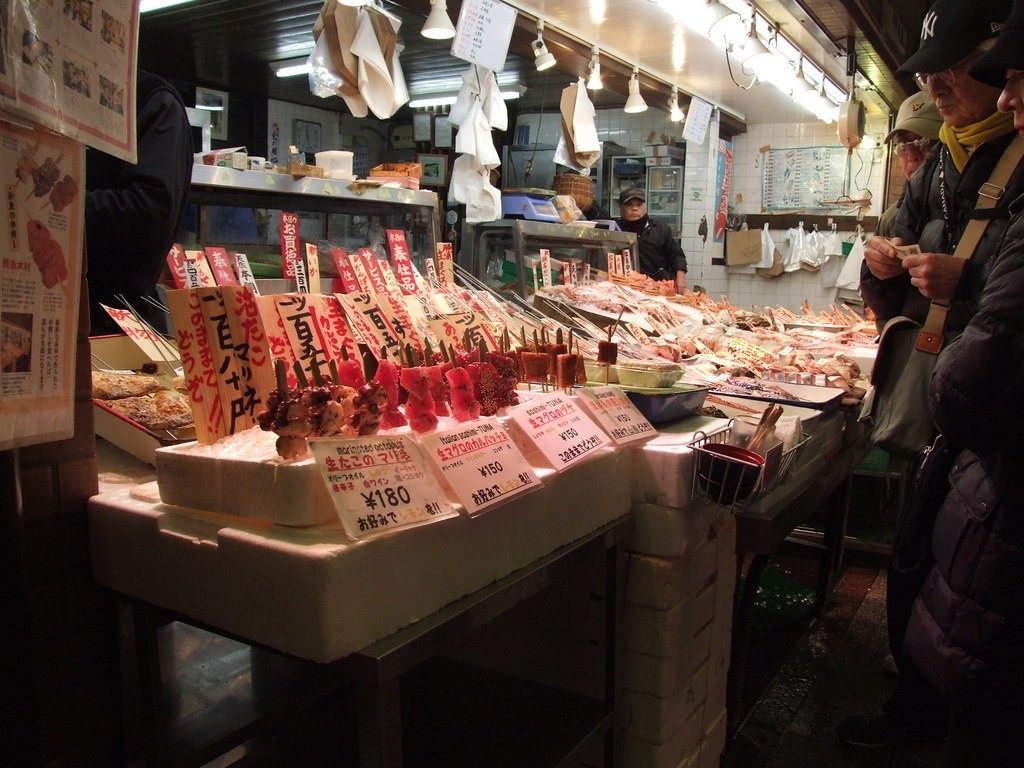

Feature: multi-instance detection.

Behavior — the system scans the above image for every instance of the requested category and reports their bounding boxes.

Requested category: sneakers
[836,706,951,748]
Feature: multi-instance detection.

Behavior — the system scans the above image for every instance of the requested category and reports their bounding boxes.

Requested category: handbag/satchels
[857,316,960,460]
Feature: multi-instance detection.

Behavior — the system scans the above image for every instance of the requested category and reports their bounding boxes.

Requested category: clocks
[292,118,322,154]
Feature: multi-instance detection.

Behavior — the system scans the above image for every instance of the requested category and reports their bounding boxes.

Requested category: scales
[820,93,871,206]
[501,187,561,222]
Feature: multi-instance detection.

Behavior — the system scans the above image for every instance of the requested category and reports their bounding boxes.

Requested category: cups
[729,415,776,455]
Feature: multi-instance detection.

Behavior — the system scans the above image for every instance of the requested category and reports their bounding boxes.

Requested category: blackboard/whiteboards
[450,0,518,73]
[682,96,713,146]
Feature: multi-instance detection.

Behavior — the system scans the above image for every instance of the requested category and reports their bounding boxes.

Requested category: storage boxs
[614,164,645,176]
[87,390,847,768]
[646,144,685,167]
[315,150,354,181]
[194,146,248,170]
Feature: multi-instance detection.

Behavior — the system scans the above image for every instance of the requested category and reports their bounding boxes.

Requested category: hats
[620,188,645,203]
[969,0,1024,89]
[883,90,945,144]
[894,0,1013,81]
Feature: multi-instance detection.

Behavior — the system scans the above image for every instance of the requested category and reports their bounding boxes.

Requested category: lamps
[421,1,457,40]
[586,45,603,90]
[667,85,684,122]
[530,19,557,71]
[705,0,840,123]
[624,66,648,114]
[407,85,528,107]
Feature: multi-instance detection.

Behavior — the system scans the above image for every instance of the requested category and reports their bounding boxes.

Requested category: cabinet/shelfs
[648,166,684,232]
[611,156,648,217]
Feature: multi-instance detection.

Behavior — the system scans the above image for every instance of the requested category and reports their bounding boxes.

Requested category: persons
[599,186,688,295]
[860,3,1013,747]
[899,1,1024,767]
[874,91,946,339]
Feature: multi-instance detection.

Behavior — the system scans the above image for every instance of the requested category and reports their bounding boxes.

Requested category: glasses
[913,50,984,94]
[893,140,936,156]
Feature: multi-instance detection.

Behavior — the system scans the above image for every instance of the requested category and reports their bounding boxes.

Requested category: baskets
[551,174,593,212]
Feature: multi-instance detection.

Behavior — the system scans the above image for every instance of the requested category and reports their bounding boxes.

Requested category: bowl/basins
[315,150,354,179]
[699,443,765,506]
[583,361,618,385]
[610,364,686,388]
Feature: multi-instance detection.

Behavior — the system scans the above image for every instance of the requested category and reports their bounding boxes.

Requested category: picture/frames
[417,154,448,186]
[195,86,229,142]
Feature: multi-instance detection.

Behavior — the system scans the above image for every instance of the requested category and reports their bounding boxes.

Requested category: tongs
[747,402,784,453]
